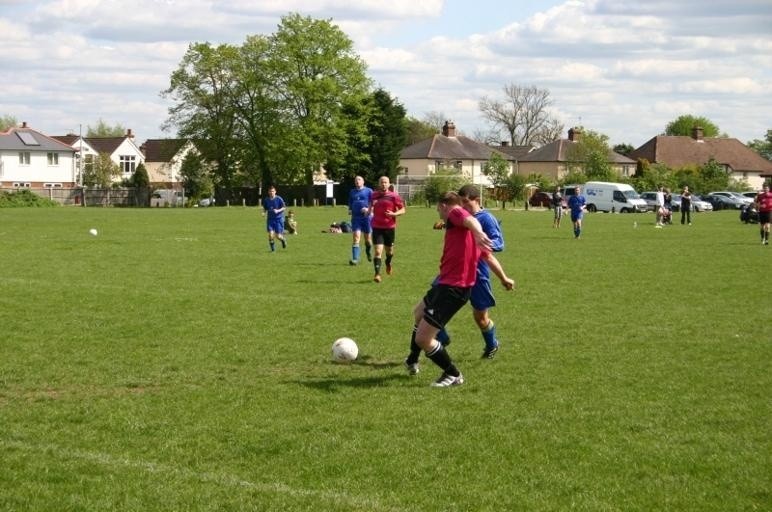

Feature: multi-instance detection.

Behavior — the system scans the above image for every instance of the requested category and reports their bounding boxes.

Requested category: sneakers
[385,260,392,274]
[482,341,498,358]
[403,356,418,375]
[374,275,382,282]
[431,371,464,387]
[283,238,287,248]
[368,253,373,261]
[349,260,358,265]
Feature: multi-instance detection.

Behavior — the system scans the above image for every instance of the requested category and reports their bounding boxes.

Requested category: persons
[367,176,406,283]
[348,175,374,265]
[553,185,565,228]
[757,182,772,245]
[654,184,692,228]
[262,186,287,251]
[284,209,297,235]
[402,191,515,389]
[431,185,502,359]
[564,186,587,240]
[744,196,760,224]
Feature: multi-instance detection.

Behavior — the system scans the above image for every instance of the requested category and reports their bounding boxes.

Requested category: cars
[151,190,215,208]
[530,192,554,207]
[640,191,762,212]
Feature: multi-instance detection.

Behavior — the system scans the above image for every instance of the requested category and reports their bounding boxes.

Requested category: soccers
[89,229,98,237]
[332,338,358,363]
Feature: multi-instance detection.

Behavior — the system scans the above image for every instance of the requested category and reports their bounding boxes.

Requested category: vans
[559,186,575,209]
[583,181,648,214]
